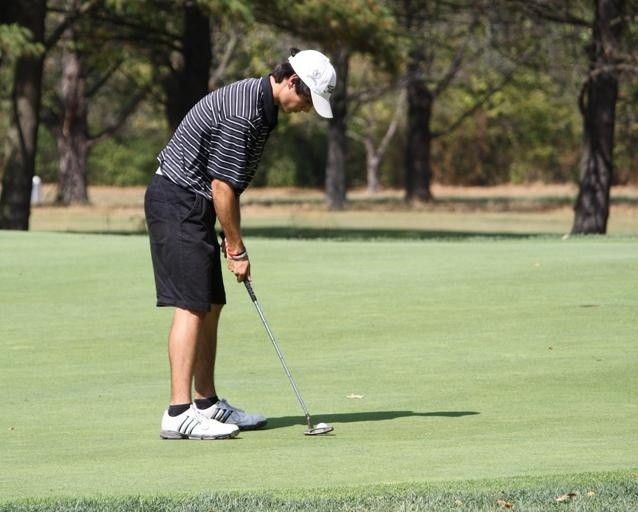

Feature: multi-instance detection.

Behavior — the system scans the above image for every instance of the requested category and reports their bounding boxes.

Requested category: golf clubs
[220,231,334,435]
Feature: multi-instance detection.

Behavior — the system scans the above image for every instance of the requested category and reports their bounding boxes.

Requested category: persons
[144,48,337,442]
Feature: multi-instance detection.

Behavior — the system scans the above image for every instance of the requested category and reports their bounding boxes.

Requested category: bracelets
[229,252,247,259]
[227,248,244,257]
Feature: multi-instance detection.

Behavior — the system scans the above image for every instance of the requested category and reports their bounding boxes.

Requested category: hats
[287,49,336,121]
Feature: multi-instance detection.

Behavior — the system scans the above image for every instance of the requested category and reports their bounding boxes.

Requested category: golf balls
[317,423,327,428]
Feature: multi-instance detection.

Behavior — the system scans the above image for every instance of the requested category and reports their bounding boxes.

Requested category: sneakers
[158,402,242,441]
[192,398,267,431]
